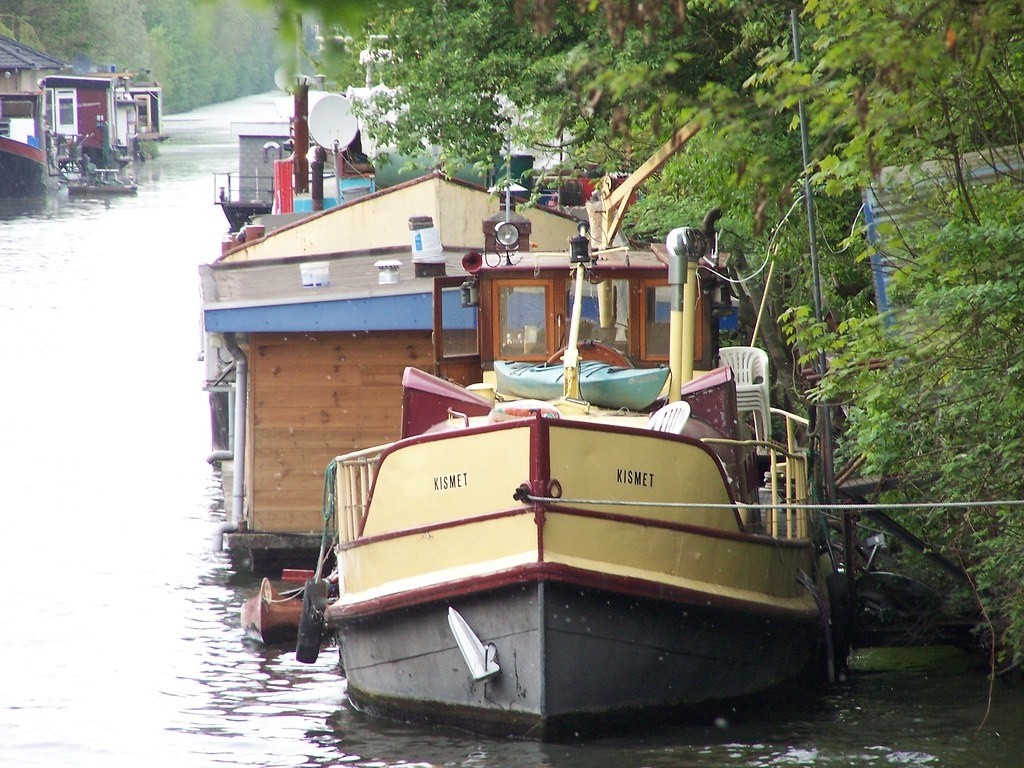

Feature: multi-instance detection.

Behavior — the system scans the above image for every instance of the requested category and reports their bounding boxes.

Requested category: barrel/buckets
[412,228,442,259]
[299,261,330,289]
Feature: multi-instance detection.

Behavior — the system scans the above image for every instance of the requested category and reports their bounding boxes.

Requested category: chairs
[718,346,771,448]
[643,401,690,434]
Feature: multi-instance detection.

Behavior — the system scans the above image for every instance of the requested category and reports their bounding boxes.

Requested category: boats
[494,355,673,415]
[240,579,306,641]
[65,168,138,194]
[287,103,852,729]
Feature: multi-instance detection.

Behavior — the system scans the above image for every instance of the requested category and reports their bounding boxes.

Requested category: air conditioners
[205,331,236,383]
